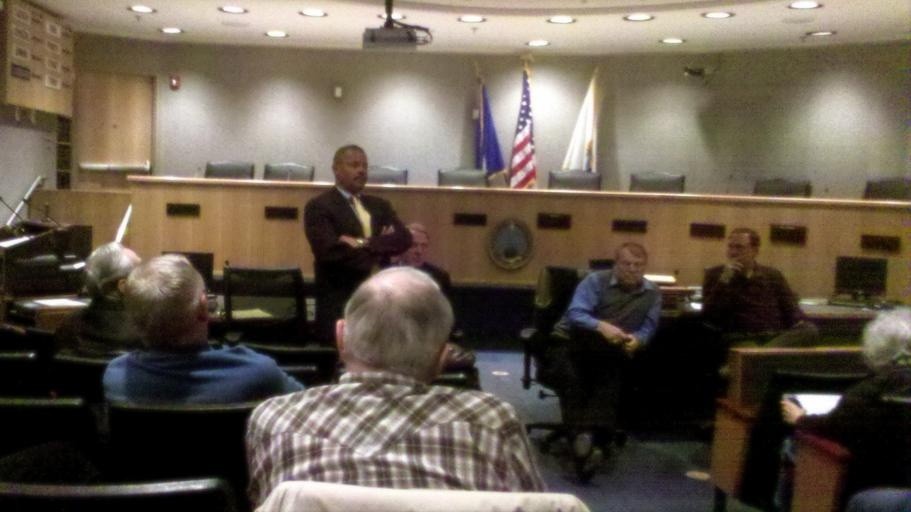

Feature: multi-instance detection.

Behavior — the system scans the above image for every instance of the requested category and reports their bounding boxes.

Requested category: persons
[779,304,911,511]
[103,251,308,404]
[244,265,571,512]
[54,241,142,359]
[546,242,662,485]
[304,145,411,375]
[698,227,818,384]
[394,222,456,322]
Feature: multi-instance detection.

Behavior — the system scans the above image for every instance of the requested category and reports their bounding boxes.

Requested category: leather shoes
[572,431,592,459]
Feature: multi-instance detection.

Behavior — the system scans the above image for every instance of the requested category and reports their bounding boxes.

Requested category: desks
[787,426,858,511]
[797,305,878,343]
[711,398,762,512]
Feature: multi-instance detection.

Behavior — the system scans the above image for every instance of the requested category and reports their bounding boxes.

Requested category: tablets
[778,390,843,424]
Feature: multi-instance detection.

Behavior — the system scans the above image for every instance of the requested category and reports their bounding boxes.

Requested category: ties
[575,448,603,486]
[351,196,372,239]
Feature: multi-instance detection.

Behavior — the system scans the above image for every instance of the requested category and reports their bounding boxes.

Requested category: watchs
[356,238,365,249]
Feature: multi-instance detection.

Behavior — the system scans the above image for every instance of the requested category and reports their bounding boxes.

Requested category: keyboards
[827,297,882,311]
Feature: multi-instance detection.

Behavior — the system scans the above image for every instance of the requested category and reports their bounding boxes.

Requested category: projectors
[360,26,418,47]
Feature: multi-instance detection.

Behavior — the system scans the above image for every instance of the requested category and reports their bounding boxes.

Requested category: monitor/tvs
[834,256,889,298]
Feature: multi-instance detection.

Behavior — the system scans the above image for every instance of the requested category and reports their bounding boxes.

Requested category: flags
[509,62,537,189]
[560,65,601,174]
[475,76,505,175]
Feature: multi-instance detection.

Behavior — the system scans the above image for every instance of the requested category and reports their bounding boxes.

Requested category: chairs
[223,267,308,343]
[0,395,106,483]
[366,166,410,185]
[733,369,873,510]
[264,162,315,182]
[548,171,603,189]
[753,180,812,197]
[0,476,235,512]
[521,264,637,459]
[204,162,254,177]
[53,352,108,397]
[1,353,42,393]
[863,177,910,199]
[161,250,214,289]
[256,480,589,512]
[106,399,255,506]
[630,172,687,191]
[439,169,488,187]
[2,323,64,362]
